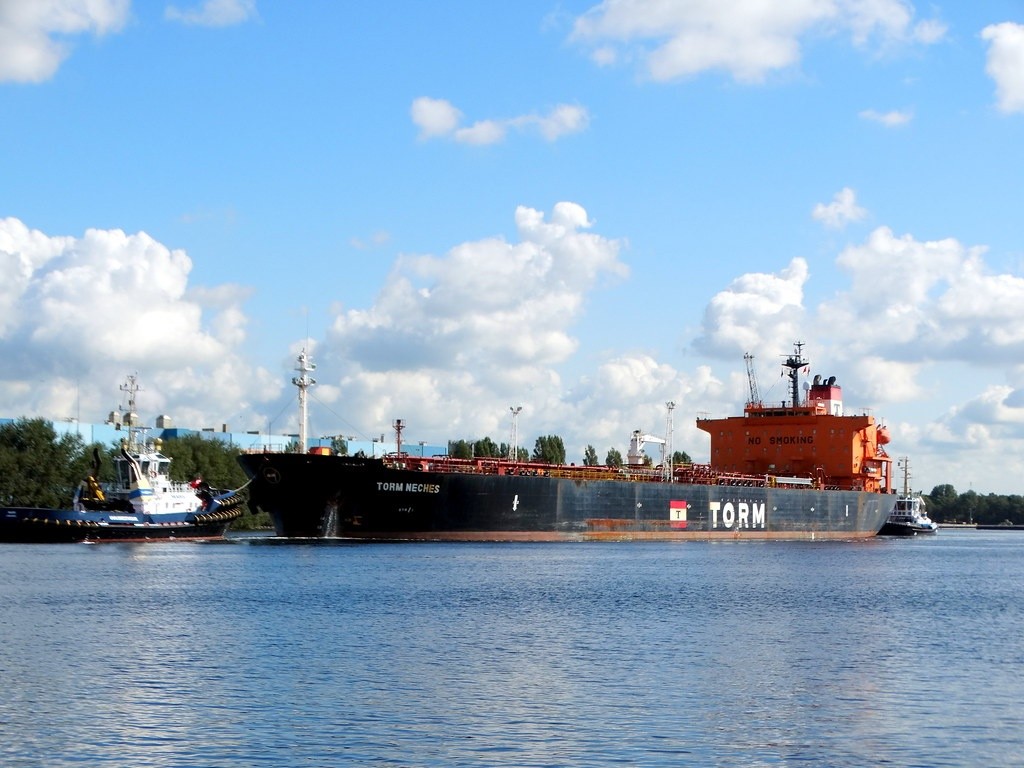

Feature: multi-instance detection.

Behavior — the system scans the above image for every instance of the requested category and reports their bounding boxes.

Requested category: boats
[882,487,939,534]
[0,375,243,540]
[232,337,901,544]
